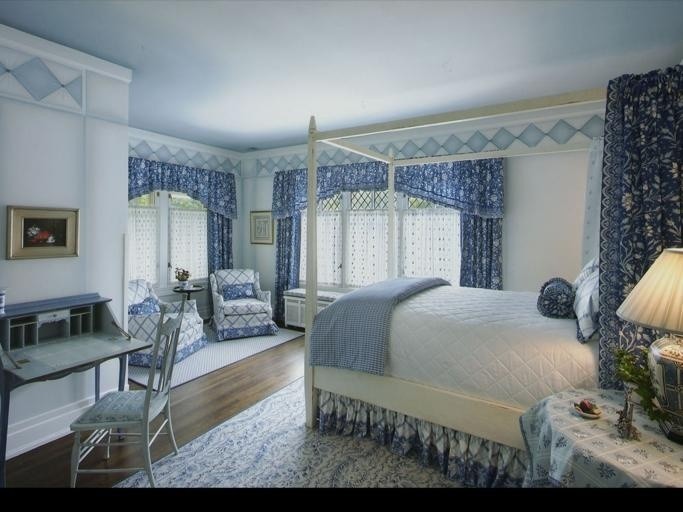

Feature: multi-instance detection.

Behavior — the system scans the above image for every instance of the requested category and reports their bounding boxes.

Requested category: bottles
[0,289,6,316]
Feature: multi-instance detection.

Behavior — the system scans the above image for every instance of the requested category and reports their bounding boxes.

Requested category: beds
[306,82,606,489]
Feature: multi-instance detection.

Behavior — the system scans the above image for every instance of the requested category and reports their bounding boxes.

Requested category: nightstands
[519,387,683,488]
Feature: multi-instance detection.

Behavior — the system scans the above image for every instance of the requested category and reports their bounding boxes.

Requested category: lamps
[617,247,683,444]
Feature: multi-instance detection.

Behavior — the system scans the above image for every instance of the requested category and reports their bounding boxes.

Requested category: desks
[0,292,153,488]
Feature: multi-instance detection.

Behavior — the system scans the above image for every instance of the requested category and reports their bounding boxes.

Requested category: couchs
[128,278,208,369]
[209,269,279,342]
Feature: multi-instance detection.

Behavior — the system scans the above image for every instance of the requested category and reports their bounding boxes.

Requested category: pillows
[128,297,161,315]
[537,255,601,343]
[222,283,257,300]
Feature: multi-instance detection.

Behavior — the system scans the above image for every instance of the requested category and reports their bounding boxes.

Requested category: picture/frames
[250,210,274,244]
[6,204,79,260]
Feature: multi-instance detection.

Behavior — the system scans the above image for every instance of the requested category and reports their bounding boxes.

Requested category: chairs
[68,300,183,487]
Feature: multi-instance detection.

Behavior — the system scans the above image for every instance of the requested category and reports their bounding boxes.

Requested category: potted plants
[175,267,191,289]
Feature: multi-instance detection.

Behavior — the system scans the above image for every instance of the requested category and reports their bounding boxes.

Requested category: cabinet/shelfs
[283,295,333,329]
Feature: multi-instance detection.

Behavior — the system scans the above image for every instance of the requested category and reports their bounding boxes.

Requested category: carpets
[114,377,483,488]
[127,322,304,392]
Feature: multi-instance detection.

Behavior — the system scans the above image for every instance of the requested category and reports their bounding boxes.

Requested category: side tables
[173,285,204,300]
